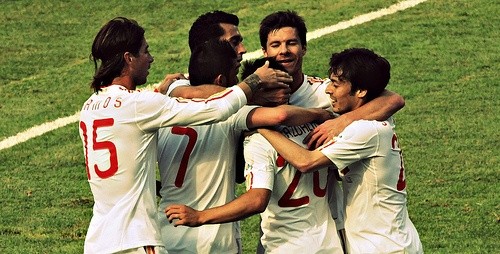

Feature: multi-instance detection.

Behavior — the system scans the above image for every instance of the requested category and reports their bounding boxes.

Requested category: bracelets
[243,74,264,98]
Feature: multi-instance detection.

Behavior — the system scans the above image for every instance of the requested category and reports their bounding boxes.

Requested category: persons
[156,39,336,254]
[165,10,247,184]
[79,16,293,254]
[243,48,424,254]
[164,56,344,254]
[257,9,405,254]
